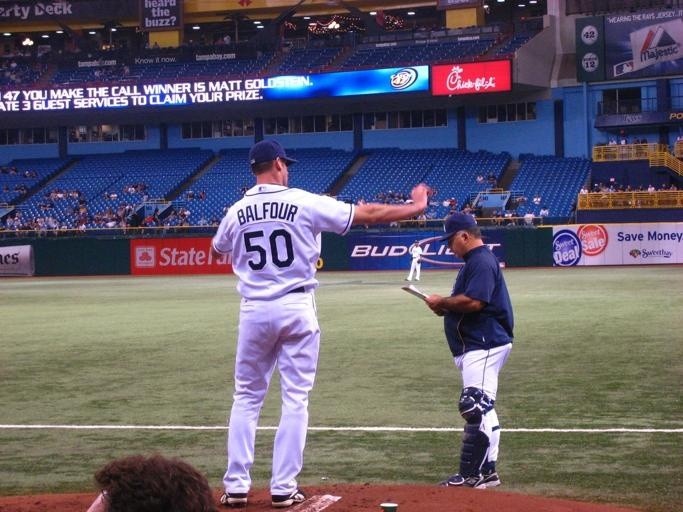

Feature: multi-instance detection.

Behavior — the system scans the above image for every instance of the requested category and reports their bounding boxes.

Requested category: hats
[249,139,300,168]
[439,213,478,242]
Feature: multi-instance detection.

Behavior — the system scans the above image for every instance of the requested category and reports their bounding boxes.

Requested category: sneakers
[271,491,306,507]
[220,492,248,507]
[439,473,502,489]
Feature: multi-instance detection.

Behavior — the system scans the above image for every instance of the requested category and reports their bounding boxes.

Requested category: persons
[424,215,515,489]
[211,139,431,508]
[476,172,496,184]
[356,191,548,228]
[580,181,678,205]
[0,166,249,238]
[405,240,423,280]
[85,455,217,511]
[608,136,648,145]
